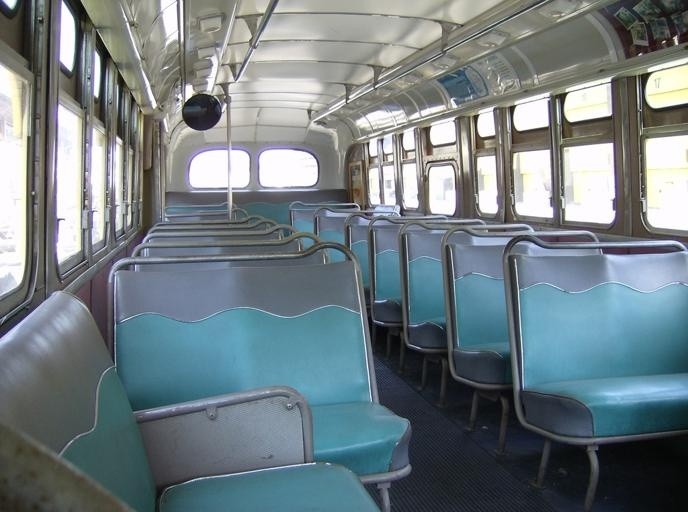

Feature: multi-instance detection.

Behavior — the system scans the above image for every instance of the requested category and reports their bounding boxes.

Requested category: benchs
[103,240,416,510]
[137,222,299,245]
[439,224,611,458]
[363,214,491,383]
[142,201,279,227]
[2,286,380,512]
[310,205,406,265]
[284,199,363,250]
[500,232,687,502]
[127,230,332,266]
[394,218,538,409]
[340,211,450,289]
